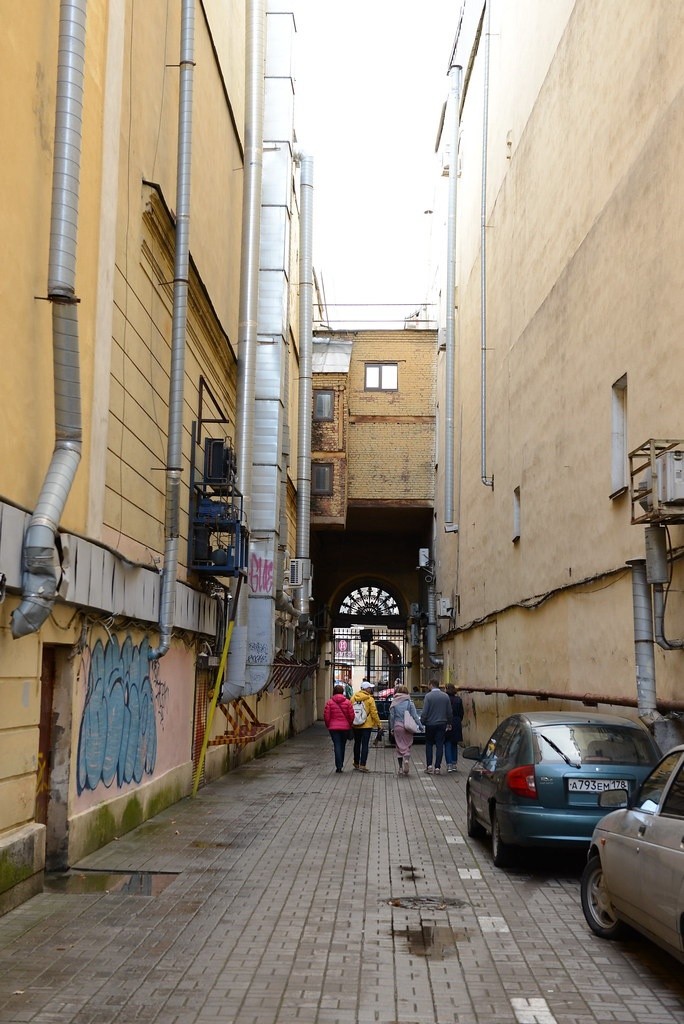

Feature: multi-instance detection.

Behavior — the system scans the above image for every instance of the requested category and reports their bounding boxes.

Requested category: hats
[361,682,375,690]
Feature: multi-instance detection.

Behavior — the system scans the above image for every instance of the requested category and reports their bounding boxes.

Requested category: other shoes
[434,768,440,775]
[453,765,458,771]
[359,765,369,772]
[352,759,359,768]
[447,764,453,773]
[398,768,403,773]
[336,767,341,773]
[403,759,409,773]
[424,765,433,773]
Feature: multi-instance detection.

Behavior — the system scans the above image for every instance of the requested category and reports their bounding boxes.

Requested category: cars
[463,711,684,961]
[390,693,430,743]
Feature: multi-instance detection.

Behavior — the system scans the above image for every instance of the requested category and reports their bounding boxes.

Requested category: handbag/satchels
[347,729,354,740]
[403,700,418,734]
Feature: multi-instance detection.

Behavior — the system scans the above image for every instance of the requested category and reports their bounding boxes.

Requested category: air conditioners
[418,548,429,567]
[637,450,684,512]
[436,597,451,617]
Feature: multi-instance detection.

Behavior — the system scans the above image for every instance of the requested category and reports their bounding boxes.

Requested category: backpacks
[352,701,370,726]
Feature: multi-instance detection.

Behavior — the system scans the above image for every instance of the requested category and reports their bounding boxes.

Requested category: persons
[389,686,424,775]
[443,683,464,773]
[346,679,353,700]
[349,682,382,772]
[419,679,453,775]
[324,685,355,773]
